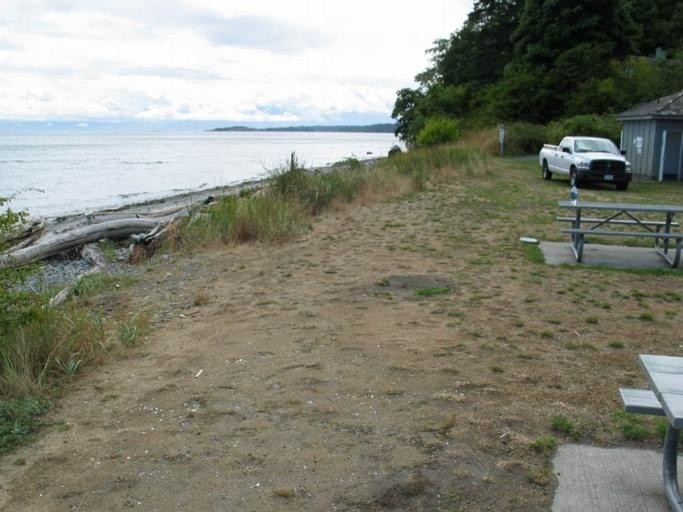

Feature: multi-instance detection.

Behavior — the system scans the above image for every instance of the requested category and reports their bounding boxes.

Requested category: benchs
[560,228,683,267]
[556,216,680,242]
[619,387,683,512]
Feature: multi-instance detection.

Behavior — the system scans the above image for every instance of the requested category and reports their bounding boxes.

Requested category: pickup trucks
[538,135,632,191]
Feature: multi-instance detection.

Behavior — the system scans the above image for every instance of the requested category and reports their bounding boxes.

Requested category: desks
[558,200,683,249]
[638,354,683,430]
